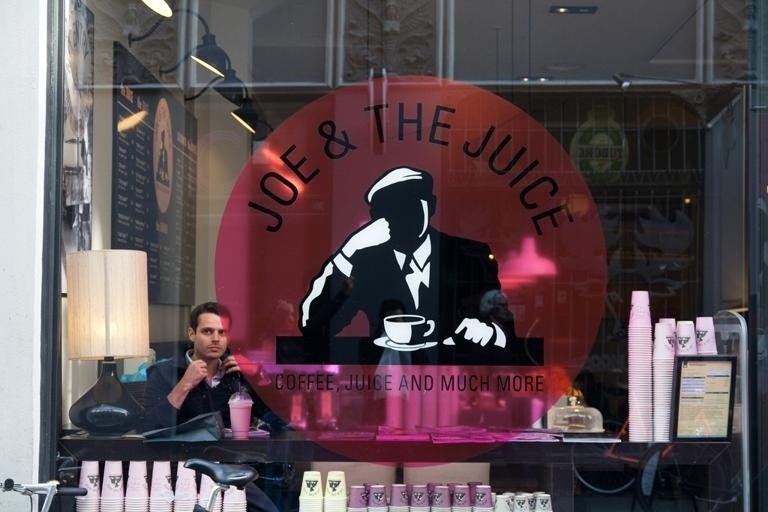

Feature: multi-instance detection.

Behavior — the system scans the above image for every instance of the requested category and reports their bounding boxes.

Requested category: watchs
[249,365,266,385]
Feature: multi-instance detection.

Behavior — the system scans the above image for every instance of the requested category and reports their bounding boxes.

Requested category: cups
[626,291,718,443]
[384,315,435,344]
[227,391,254,432]
[74,461,248,511]
[298,470,554,512]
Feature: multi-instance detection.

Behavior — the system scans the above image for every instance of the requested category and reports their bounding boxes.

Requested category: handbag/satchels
[122,411,224,444]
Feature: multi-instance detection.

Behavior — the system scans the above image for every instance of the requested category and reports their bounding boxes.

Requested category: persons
[353,0,432,71]
[297,167,515,349]
[371,298,426,364]
[464,289,510,365]
[145,303,290,438]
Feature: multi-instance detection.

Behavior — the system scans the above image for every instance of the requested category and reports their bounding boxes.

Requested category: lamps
[127,0,259,136]
[66,250,151,436]
[612,72,742,92]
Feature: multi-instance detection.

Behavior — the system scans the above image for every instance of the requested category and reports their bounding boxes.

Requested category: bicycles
[1,457,258,510]
[572,373,707,493]
[635,414,768,512]
[60,445,298,509]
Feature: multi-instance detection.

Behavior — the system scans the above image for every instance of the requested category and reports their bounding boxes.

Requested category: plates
[373,336,438,352]
